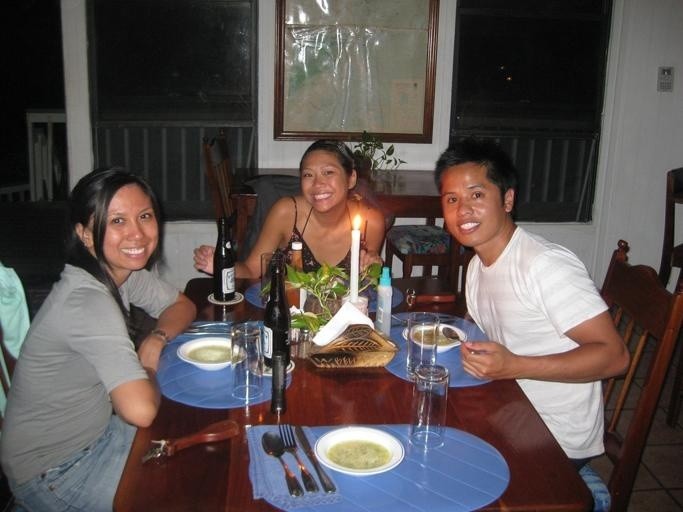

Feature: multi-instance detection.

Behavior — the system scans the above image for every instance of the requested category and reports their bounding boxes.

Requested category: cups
[229,319,267,399]
[410,364,448,449]
[261,251,286,295]
[403,309,438,376]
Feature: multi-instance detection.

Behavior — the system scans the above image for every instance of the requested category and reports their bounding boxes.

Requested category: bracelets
[149,328,171,346]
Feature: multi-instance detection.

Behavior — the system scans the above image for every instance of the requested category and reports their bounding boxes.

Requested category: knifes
[293,422,338,496]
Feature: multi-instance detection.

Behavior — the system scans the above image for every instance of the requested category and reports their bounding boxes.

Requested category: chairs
[201,128,237,245]
[0,261,29,510]
[654,166,683,301]
[577,237,681,512]
[384,224,475,293]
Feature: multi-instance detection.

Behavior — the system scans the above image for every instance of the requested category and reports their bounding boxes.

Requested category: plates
[400,322,467,357]
[176,337,244,373]
[312,426,404,478]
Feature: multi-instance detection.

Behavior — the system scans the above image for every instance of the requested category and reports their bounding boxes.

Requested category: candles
[346,215,364,300]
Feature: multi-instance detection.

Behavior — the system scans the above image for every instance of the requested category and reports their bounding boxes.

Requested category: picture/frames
[271,0,441,146]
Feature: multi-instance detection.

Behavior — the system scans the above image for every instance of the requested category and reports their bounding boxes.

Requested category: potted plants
[262,258,383,334]
[342,129,406,176]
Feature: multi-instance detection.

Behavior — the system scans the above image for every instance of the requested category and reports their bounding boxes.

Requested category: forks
[277,423,320,496]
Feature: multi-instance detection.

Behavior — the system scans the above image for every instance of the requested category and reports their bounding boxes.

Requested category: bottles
[263,267,290,370]
[271,352,287,415]
[374,264,393,337]
[296,326,310,358]
[212,216,237,301]
[290,243,304,273]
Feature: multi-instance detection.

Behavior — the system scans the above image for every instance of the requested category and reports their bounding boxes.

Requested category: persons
[0,164,199,508]
[433,136,633,475]
[191,136,389,280]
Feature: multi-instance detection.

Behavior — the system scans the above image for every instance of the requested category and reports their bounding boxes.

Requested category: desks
[229,167,466,277]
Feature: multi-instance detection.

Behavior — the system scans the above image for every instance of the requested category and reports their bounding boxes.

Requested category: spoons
[439,324,478,357]
[260,432,307,501]
